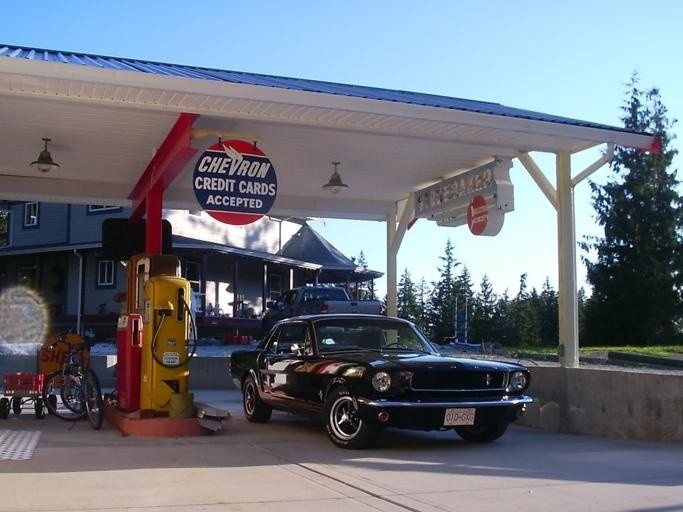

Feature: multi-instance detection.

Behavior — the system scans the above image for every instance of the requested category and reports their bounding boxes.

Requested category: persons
[248,308,256,320]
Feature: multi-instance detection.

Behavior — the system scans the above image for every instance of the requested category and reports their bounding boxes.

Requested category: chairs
[320,328,352,348]
[359,329,387,347]
[280,328,353,348]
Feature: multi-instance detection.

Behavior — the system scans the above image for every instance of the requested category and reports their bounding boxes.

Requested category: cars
[230,313,533,448]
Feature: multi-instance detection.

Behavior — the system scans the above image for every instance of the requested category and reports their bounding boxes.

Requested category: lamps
[321,161,348,194]
[30,138,61,172]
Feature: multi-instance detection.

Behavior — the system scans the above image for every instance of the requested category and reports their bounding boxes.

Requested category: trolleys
[0,371,57,418]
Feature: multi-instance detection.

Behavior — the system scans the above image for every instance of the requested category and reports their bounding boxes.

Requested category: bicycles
[41,328,104,430]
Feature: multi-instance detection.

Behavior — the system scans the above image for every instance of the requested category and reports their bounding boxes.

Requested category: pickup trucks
[260,286,384,333]
